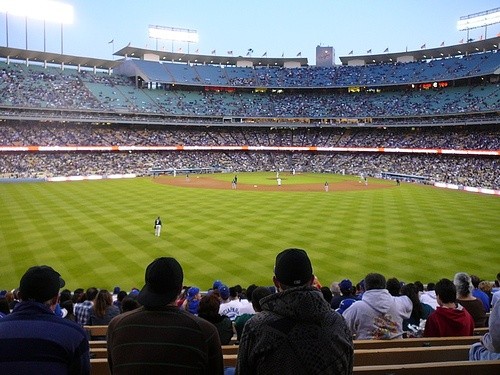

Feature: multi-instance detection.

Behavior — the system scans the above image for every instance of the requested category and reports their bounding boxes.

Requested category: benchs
[83,326,500,375]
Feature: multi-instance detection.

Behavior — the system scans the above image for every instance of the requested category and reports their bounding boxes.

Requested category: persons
[154,216,162,237]
[0,248,500,375]
[0,61,500,197]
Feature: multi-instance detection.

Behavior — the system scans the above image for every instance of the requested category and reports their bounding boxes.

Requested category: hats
[131,290,139,294]
[338,279,352,290]
[136,257,183,307]
[186,287,199,300]
[360,280,365,292]
[273,249,312,287]
[20,266,65,299]
[213,280,222,289]
[218,285,230,298]
